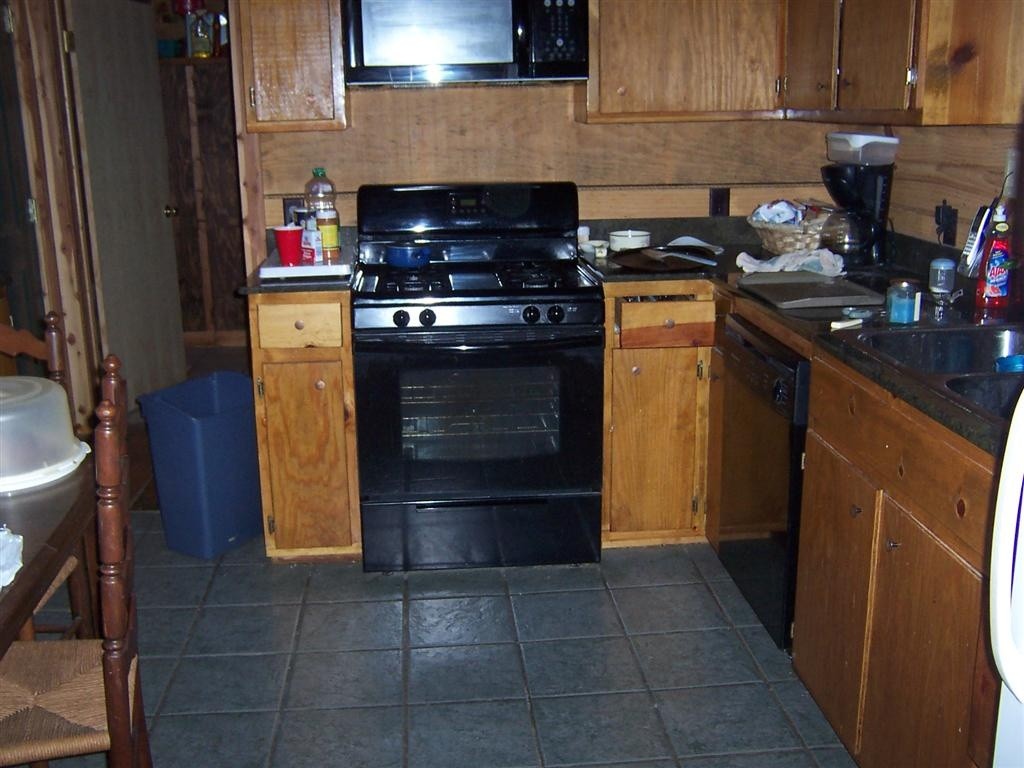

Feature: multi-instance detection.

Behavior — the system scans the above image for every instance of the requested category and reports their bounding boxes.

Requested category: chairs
[0,355,155,768]
[0,310,92,646]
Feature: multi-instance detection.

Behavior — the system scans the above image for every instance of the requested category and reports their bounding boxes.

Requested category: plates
[609,246,716,274]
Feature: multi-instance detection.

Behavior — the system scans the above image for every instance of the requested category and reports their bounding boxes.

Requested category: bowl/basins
[826,131,900,166]
[0,375,93,500]
[608,231,653,252]
[584,240,609,258]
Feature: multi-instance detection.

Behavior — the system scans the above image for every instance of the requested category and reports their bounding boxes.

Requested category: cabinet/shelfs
[571,0,1024,126]
[792,347,1001,768]
[602,280,716,551]
[248,290,362,563]
[226,0,351,132]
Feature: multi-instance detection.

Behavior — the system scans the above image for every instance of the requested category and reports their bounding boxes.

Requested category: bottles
[928,259,956,293]
[883,277,924,326]
[974,214,1017,310]
[314,210,342,261]
[190,15,212,59]
[576,223,590,245]
[304,167,337,219]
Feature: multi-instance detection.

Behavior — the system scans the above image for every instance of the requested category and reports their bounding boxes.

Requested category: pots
[384,239,431,268]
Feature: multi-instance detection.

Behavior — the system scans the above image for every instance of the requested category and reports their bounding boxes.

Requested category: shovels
[640,249,719,266]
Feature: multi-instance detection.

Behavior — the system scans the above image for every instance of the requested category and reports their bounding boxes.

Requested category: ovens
[351,324,605,575]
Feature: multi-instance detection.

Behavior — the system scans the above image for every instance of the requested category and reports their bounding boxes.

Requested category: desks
[0,432,100,655]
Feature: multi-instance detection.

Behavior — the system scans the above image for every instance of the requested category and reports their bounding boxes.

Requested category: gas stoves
[350,180,607,332]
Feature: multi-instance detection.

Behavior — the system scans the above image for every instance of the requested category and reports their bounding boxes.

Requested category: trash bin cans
[139,369,262,560]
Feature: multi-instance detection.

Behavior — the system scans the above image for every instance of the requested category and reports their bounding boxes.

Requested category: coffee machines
[819,163,903,274]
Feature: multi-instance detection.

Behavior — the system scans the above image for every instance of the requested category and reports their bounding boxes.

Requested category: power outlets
[943,209,957,246]
[283,198,303,224]
[709,187,730,216]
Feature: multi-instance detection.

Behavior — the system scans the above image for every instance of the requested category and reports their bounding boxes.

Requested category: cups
[293,208,316,227]
[272,226,305,266]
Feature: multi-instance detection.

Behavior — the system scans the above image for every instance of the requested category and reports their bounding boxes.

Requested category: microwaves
[336,0,592,91]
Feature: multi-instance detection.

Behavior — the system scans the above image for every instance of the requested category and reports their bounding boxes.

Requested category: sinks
[945,376,1023,422]
[859,331,1024,370]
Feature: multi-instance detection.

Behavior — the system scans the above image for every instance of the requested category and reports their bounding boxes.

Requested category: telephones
[958,198,998,278]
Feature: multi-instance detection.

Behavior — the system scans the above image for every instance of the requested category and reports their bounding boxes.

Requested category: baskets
[746,210,828,256]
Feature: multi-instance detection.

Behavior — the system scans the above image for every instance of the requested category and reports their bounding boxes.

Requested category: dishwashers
[706,315,818,657]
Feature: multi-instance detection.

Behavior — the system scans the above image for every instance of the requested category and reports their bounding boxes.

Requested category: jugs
[820,210,877,255]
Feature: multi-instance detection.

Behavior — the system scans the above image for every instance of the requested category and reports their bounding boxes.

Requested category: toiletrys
[975,205,1011,310]
